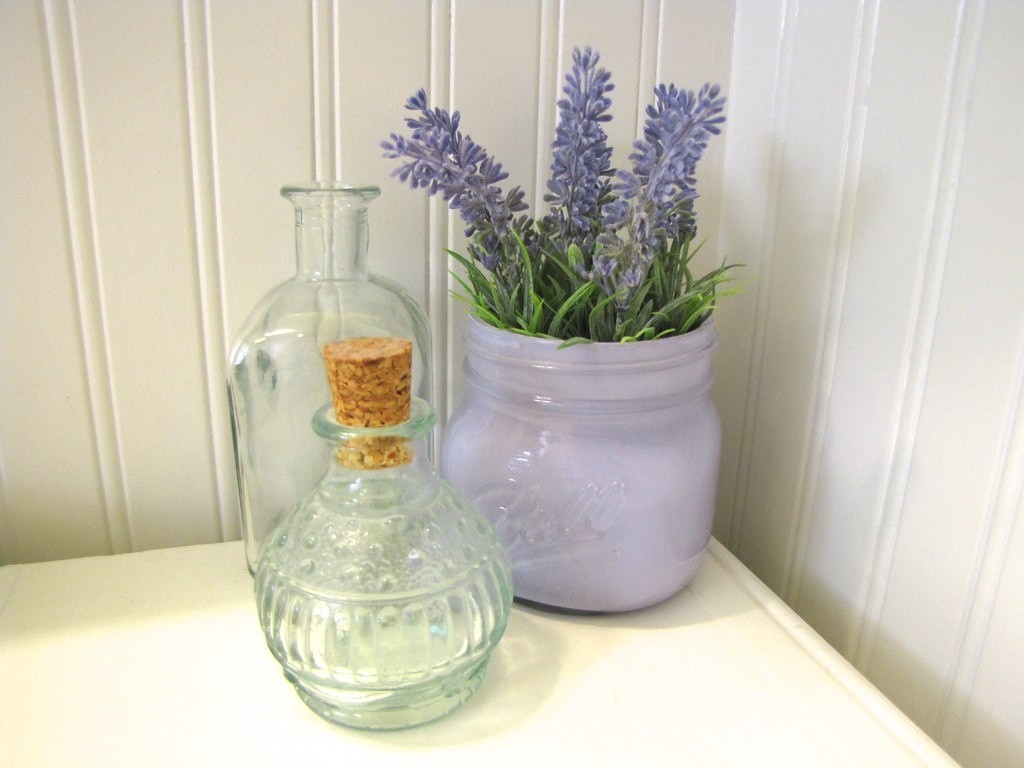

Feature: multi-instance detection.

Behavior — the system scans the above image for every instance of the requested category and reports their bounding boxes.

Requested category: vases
[438,310,723,614]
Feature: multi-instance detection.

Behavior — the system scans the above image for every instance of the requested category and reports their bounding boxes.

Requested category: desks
[0,536,958,768]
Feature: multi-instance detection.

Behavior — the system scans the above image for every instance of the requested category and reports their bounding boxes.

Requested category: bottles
[226,186,433,581]
[253,337,512,729]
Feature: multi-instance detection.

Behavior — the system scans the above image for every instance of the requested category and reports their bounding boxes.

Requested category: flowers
[376,47,750,350]
